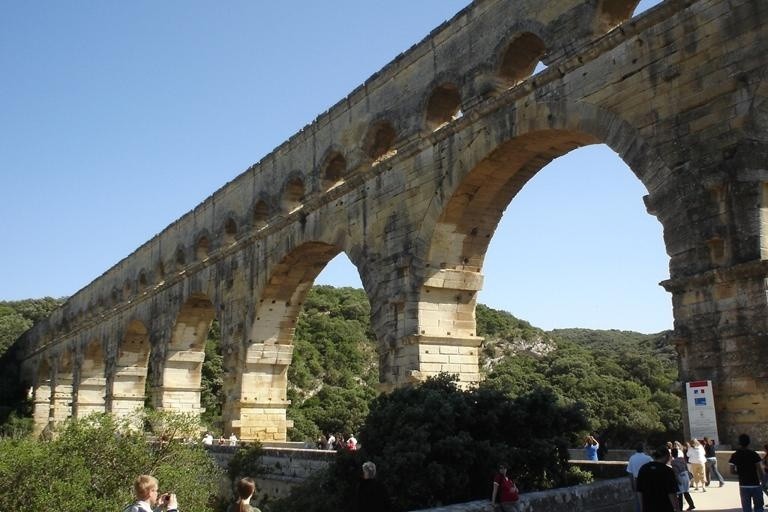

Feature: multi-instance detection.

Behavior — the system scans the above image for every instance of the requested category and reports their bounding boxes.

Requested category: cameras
[165,494,170,501]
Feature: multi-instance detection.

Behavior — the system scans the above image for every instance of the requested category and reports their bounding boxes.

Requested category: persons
[301,432,358,452]
[351,461,391,512]
[122,474,179,512]
[229,432,237,446]
[227,477,260,512]
[579,432,768,512]
[488,461,525,512]
[207,432,214,442]
[201,430,212,445]
[217,432,226,446]
